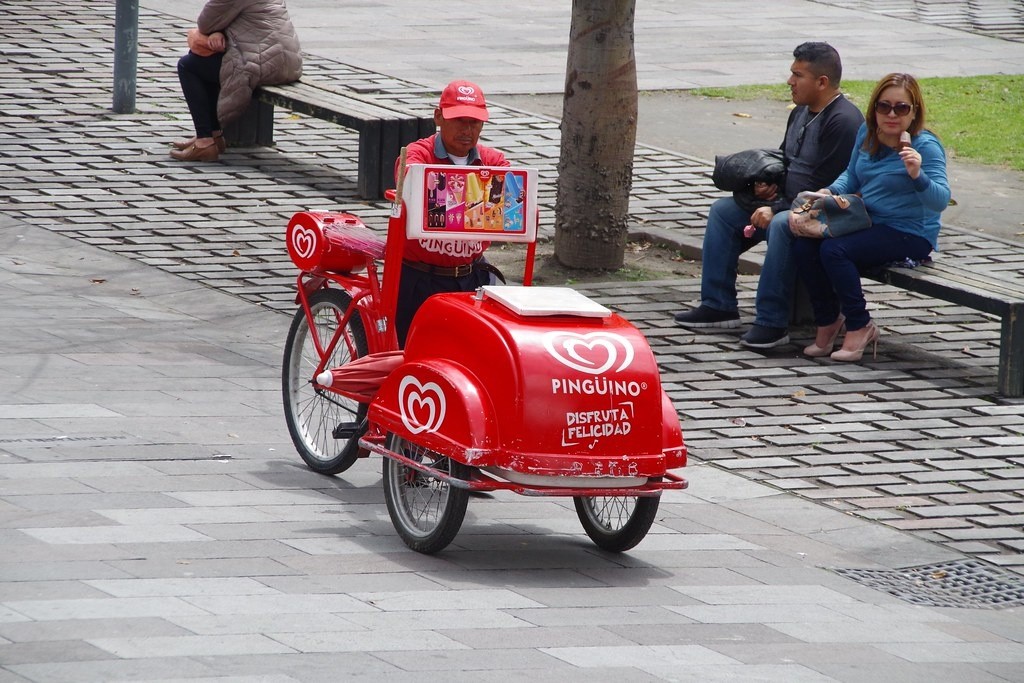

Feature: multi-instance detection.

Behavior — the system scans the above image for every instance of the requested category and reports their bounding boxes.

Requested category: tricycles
[285,190,691,556]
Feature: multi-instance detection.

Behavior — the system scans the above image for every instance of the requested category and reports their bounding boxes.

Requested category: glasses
[875,101,914,116]
[793,126,806,157]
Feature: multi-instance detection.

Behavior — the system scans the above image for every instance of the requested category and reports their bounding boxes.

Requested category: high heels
[804,313,846,356]
[830,320,880,362]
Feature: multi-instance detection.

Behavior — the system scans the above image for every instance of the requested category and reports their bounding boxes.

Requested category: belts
[402,255,507,285]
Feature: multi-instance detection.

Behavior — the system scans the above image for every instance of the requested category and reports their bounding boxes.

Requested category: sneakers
[674,304,742,328]
[740,323,790,348]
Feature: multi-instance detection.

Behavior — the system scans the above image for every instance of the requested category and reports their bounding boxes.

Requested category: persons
[675,43,865,347]
[396,80,513,486]
[169,0,303,163]
[803,73,952,361]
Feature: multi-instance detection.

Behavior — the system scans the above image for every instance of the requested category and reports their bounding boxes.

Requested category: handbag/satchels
[788,190,871,238]
[188,29,216,57]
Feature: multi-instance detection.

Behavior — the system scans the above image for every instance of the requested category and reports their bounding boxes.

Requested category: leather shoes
[170,142,219,162]
[173,136,226,153]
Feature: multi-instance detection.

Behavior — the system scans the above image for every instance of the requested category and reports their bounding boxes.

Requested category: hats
[440,80,489,122]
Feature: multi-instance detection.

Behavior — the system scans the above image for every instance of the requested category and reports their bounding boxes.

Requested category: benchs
[219,79,436,202]
[786,251,1024,397]
